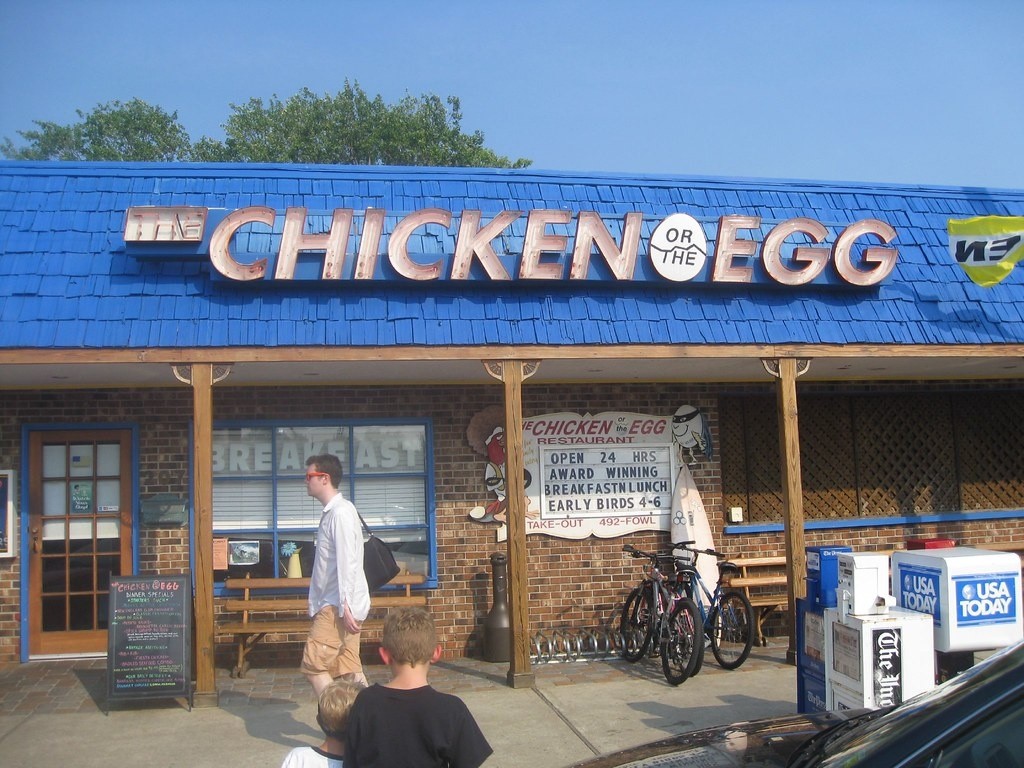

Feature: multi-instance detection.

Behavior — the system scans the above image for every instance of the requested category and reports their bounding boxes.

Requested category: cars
[571,639,1024,768]
[43,540,120,622]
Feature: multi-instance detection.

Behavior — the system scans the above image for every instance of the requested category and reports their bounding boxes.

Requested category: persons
[342,606,493,768]
[282,680,366,768]
[298,454,371,697]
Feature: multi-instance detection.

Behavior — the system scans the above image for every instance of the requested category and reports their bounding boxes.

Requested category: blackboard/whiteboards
[108,574,192,701]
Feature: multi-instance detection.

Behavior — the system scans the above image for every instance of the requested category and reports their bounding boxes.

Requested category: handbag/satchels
[358,511,401,593]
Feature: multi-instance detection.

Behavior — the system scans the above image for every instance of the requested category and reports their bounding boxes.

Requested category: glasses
[306,473,325,480]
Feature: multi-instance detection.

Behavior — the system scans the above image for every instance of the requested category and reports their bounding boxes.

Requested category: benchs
[958,540,1024,592]
[716,549,909,647]
[218,570,427,679]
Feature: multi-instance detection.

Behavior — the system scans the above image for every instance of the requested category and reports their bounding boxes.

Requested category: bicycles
[620,541,754,685]
